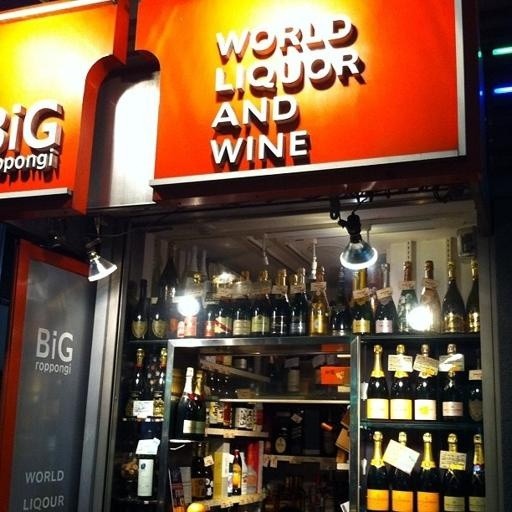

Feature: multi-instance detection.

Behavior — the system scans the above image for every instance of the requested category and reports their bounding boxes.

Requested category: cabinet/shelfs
[103,331,486,512]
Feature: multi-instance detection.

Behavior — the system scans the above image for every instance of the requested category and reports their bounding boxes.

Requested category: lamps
[84,218,117,282]
[327,190,378,270]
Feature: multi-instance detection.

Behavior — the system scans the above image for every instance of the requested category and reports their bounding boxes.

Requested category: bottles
[120,342,354,511]
[358,342,483,422]
[361,422,486,512]
[120,255,485,341]
